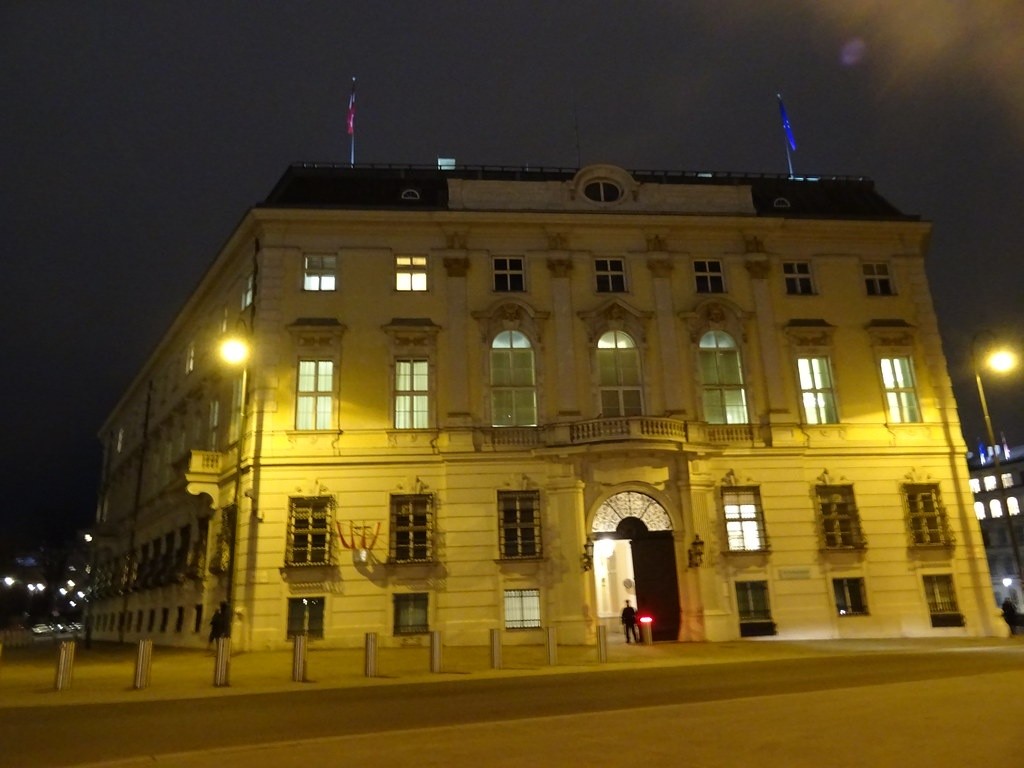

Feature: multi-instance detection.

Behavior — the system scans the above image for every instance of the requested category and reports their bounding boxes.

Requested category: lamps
[581,536,593,570]
[689,533,706,568]
[360,547,370,564]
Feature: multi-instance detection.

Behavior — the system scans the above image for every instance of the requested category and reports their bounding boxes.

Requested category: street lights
[971,329,1023,590]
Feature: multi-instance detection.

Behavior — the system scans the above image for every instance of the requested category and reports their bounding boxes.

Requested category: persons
[203,608,223,657]
[621,600,638,643]
[1002,598,1017,635]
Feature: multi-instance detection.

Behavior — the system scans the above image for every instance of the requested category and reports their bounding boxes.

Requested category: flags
[780,102,796,152]
[347,82,356,134]
